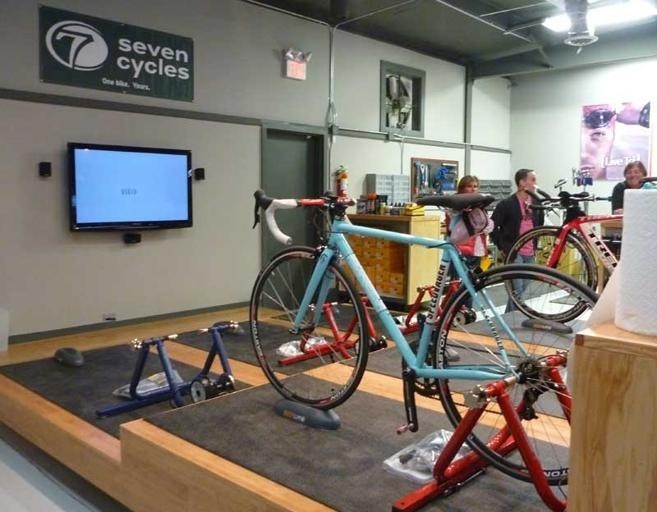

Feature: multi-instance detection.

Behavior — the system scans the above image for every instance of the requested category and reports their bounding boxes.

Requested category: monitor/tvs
[66,141,194,233]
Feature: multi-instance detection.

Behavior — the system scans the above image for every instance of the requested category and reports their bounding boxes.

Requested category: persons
[447,174,487,324]
[611,160,657,214]
[487,169,544,313]
[580,102,649,179]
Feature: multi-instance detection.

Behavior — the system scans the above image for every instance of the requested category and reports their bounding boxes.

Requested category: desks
[566,319,657,512]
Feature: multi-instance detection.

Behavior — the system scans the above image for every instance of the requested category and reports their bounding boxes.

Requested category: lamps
[283,48,312,81]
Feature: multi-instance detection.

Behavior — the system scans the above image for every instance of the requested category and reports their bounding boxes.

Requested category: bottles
[356,193,406,214]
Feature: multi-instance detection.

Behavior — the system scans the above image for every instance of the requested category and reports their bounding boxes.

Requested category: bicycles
[250,188,600,485]
[504,175,657,323]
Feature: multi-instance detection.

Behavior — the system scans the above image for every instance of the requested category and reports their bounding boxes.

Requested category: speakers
[195,167,205,180]
[39,162,52,177]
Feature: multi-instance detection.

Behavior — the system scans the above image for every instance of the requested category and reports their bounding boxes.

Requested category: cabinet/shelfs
[336,215,441,311]
[600,219,623,296]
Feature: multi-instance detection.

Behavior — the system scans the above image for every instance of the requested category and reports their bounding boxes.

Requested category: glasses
[582,109,616,127]
